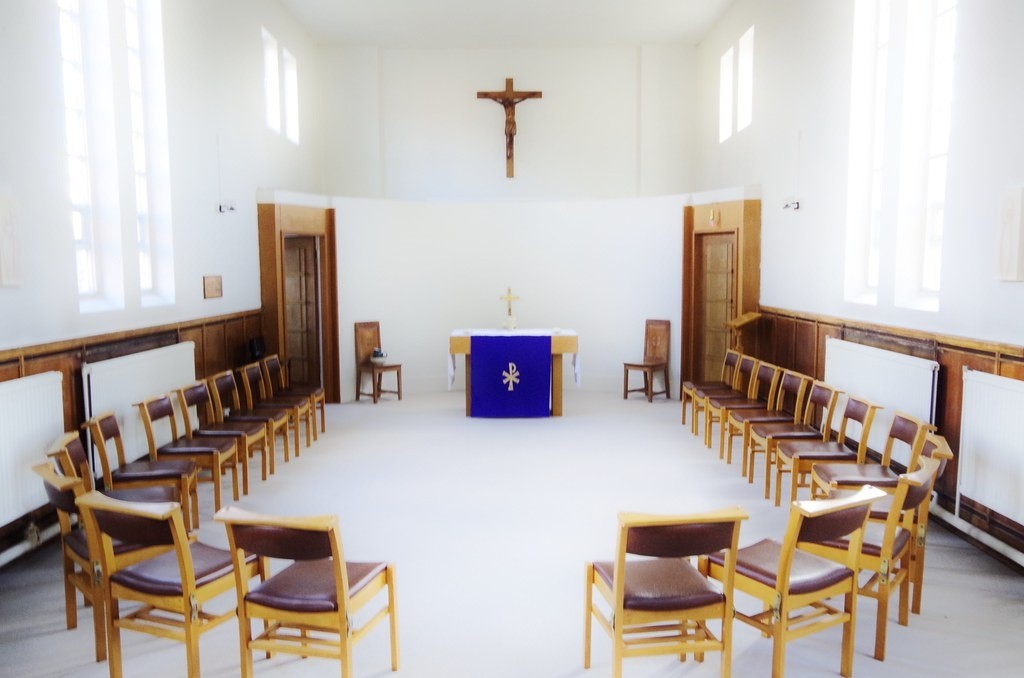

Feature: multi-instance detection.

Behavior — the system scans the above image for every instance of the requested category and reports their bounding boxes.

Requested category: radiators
[0,369,78,567]
[954,364,1024,530]
[79,340,196,482]
[823,334,940,496]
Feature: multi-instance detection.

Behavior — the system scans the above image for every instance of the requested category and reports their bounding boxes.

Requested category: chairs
[354,322,402,404]
[584,504,747,678]
[134,353,325,514]
[75,490,275,678]
[33,462,198,662]
[793,456,943,663]
[694,485,888,678]
[79,410,200,541]
[683,350,936,512]
[624,319,670,403]
[45,432,185,606]
[213,505,399,678]
[827,432,950,613]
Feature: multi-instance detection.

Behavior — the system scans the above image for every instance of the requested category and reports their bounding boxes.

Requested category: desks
[447,330,582,418]
